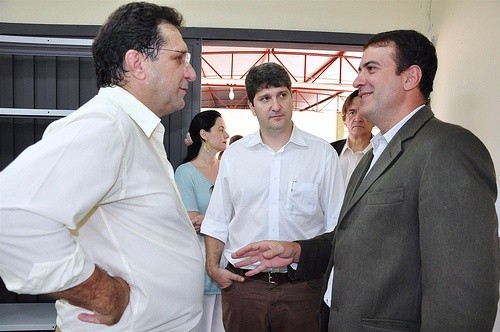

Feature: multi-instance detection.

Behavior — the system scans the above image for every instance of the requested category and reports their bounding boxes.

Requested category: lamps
[229,87,234,100]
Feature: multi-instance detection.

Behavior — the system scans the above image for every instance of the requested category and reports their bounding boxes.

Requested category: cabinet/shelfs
[0,34,97,332]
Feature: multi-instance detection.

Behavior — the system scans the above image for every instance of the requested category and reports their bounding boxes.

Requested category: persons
[330,90,375,187]
[231,30,500,332]
[200,62,346,332]
[174,110,244,332]
[0,2,205,332]
[218,135,243,160]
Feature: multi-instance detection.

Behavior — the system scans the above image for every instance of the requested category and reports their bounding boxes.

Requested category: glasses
[143,47,193,68]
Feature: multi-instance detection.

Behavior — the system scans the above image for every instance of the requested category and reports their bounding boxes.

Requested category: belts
[225,261,292,284]
